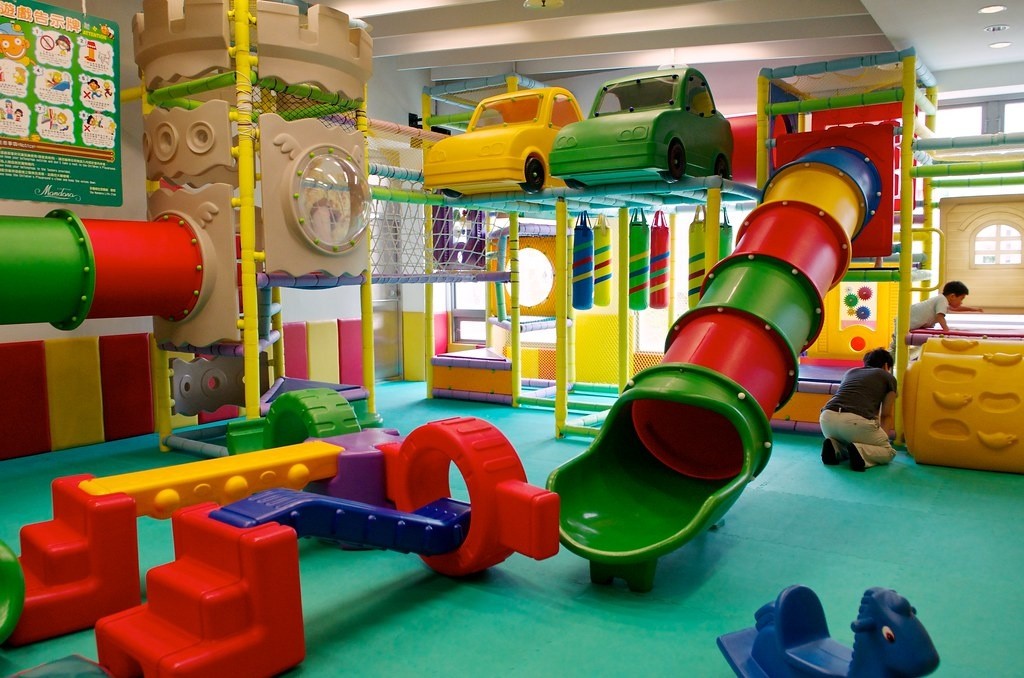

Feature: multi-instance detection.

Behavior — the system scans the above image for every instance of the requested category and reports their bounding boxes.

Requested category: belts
[828,405,854,414]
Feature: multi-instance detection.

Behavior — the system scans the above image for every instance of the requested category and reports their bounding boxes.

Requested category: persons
[820,349,900,472]
[887,280,971,367]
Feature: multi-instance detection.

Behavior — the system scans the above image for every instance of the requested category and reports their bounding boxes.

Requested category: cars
[419,86,587,199]
[546,67,735,188]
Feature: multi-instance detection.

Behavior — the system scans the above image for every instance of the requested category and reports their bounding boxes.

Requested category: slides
[544,141,882,593]
